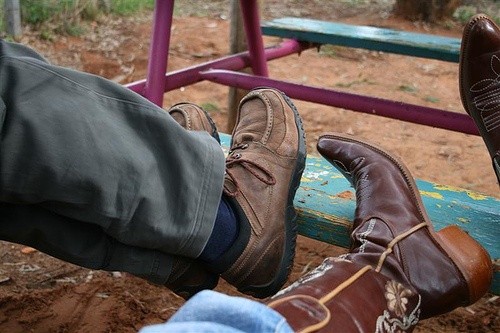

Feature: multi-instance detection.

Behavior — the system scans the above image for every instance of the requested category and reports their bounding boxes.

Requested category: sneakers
[162,101,221,301]
[219,86,308,300]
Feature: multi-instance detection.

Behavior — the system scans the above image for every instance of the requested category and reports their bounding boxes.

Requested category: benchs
[206,130,500,297]
[259,17,462,62]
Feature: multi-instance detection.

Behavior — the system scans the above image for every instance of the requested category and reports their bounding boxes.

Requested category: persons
[0,40,307,302]
[135,13,500,333]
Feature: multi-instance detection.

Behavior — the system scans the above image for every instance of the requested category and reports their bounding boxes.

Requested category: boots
[265,132,493,333]
[459,13,500,186]
